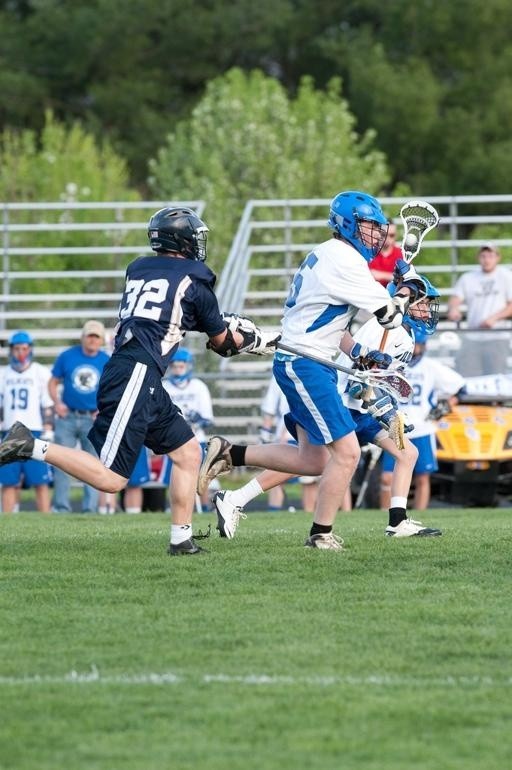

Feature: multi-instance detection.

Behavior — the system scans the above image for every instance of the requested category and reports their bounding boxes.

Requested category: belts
[70,408,98,414]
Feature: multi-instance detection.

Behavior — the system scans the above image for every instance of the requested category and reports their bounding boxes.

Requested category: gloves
[368,396,414,433]
[392,259,428,308]
[206,312,281,358]
[186,410,212,428]
[350,343,392,369]
[428,400,452,420]
[39,431,54,442]
[258,426,273,444]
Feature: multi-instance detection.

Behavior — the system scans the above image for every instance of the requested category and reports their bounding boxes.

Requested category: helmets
[169,350,193,385]
[8,333,34,373]
[327,190,390,262]
[147,207,210,263]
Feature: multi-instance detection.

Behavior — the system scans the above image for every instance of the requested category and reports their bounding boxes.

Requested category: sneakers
[385,516,442,537]
[0,421,35,467]
[167,524,212,556]
[304,530,344,550]
[197,436,233,496]
[212,490,247,539]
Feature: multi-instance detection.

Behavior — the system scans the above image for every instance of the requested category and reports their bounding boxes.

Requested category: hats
[82,320,106,339]
[481,241,499,254]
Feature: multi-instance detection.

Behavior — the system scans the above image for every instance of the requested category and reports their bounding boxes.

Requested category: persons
[213,273,441,539]
[0,329,56,511]
[160,348,215,513]
[367,218,402,289]
[259,370,308,511]
[97,442,152,514]
[379,327,468,511]
[198,191,416,549]
[0,206,281,556]
[447,242,512,329]
[47,320,112,514]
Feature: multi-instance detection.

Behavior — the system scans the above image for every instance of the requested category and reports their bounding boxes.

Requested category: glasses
[388,233,395,238]
